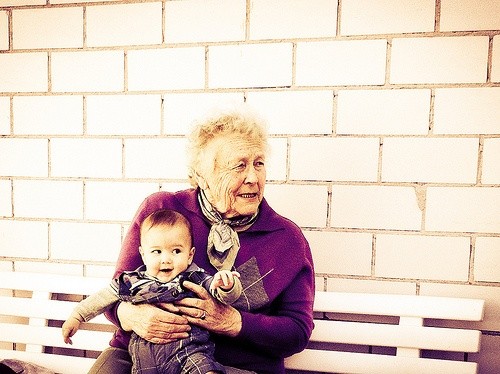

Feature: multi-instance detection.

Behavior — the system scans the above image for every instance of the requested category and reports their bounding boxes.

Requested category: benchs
[0,270,485,374]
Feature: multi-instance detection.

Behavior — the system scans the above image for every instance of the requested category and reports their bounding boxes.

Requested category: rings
[201,311,206,320]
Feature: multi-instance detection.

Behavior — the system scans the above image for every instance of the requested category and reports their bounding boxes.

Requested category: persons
[88,114,315,374]
[62,210,243,374]
